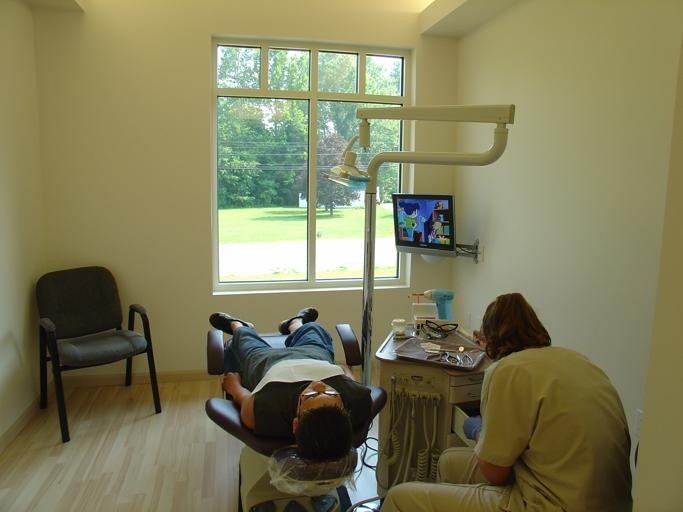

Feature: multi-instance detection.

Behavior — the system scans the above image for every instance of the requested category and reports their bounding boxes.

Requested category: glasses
[298,391,345,421]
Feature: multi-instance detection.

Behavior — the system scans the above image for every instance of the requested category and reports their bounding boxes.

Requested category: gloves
[463,414,483,440]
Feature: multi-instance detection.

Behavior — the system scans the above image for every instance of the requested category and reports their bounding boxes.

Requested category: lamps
[324,102,515,385]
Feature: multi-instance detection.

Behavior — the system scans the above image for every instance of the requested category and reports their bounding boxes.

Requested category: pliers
[426,352,474,366]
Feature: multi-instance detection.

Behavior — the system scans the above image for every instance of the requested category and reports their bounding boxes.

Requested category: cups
[391,319,406,339]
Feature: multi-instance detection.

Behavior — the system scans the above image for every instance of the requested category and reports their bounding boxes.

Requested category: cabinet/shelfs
[374,324,496,499]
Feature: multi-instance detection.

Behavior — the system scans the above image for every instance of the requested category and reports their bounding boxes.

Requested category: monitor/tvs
[392,193,458,257]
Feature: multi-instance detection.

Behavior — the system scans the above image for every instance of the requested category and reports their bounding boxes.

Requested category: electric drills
[412,289,454,319]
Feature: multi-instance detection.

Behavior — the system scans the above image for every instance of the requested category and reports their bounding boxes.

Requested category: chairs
[35,264,160,444]
[204,323,387,498]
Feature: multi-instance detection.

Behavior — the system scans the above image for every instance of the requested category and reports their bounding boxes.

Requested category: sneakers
[278,307,318,336]
[208,312,255,335]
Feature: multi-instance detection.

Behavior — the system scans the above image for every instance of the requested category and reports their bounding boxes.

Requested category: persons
[205,306,374,466]
[372,292,634,512]
